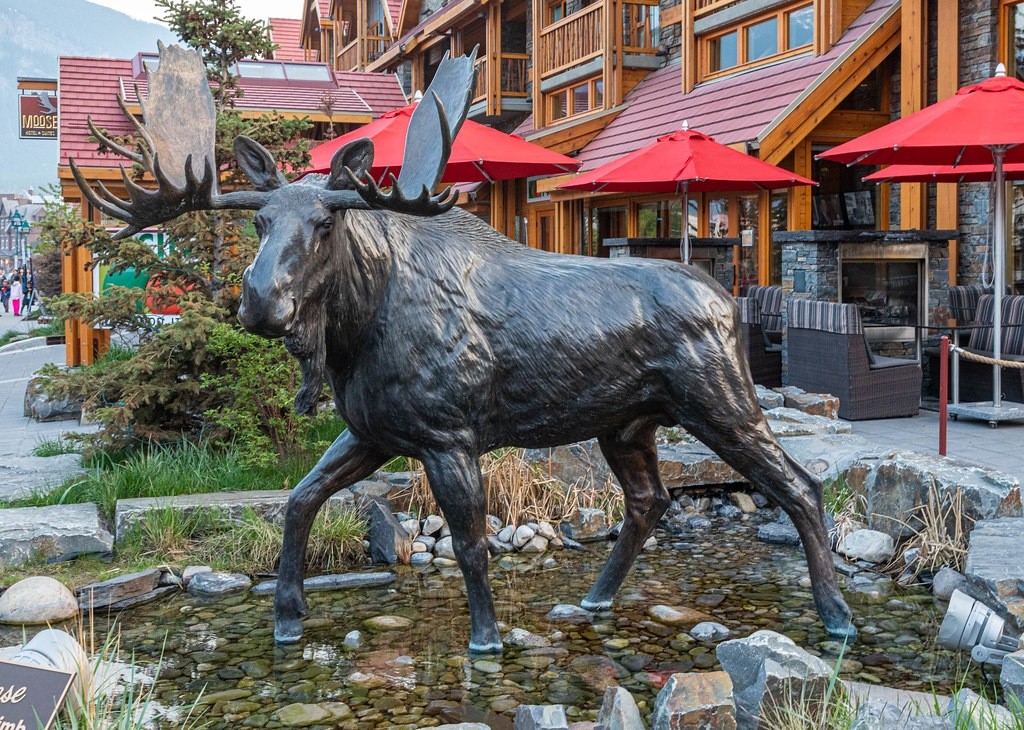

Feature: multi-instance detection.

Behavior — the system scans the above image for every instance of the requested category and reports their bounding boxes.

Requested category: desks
[874,320,1022,412]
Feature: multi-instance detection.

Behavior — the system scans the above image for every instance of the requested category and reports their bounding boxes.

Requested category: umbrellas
[815,63,1024,407]
[555,120,820,265]
[275,88,584,189]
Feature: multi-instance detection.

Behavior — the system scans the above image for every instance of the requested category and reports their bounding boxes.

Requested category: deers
[69,41,863,650]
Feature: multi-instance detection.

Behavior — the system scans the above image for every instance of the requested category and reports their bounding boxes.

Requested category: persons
[10,275,23,316]
[6,268,32,293]
[20,281,33,315]
[0,266,10,313]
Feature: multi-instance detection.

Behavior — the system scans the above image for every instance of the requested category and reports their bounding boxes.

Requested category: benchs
[786,300,923,422]
[948,285,1024,403]
[732,296,782,389]
[747,285,783,344]
